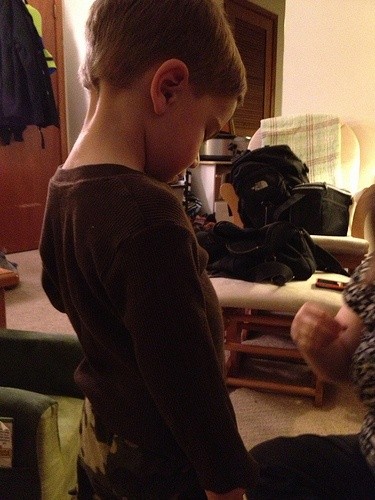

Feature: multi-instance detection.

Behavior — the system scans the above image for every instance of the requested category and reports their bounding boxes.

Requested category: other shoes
[0,251,19,289]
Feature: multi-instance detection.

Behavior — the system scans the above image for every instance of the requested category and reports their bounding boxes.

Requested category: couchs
[0,328,89,500]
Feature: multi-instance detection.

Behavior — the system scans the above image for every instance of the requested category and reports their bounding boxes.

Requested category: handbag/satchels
[272,181,355,236]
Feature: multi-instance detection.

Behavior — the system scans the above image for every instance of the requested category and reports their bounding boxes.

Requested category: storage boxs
[265,181,353,235]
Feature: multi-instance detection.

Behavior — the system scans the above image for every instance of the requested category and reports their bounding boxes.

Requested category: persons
[38,0,261,500]
[244,204,375,500]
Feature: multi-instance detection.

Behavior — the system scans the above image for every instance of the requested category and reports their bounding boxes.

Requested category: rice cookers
[200,131,237,160]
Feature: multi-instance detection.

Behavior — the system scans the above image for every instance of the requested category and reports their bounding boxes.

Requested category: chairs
[207,114,375,406]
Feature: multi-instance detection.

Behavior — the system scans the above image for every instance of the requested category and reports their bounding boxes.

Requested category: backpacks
[228,144,310,227]
